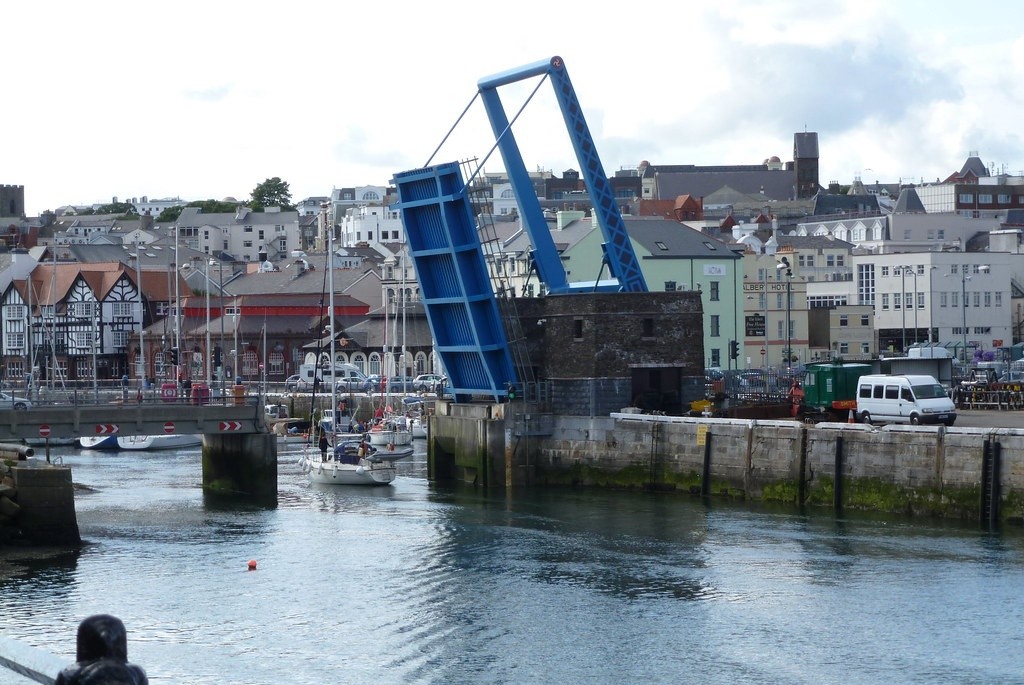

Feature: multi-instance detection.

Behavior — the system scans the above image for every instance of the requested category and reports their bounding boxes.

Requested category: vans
[857,374,957,426]
[298,362,368,390]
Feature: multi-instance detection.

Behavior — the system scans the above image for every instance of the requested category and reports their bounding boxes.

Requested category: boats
[80,434,202,451]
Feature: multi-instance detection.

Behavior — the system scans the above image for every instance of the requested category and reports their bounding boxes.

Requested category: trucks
[908,346,961,365]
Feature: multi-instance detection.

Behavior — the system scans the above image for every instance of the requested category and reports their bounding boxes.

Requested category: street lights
[962,266,990,366]
[894,265,911,353]
[765,263,787,370]
[176,263,190,386]
[907,271,917,344]
[928,266,941,342]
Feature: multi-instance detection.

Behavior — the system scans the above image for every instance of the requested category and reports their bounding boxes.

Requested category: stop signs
[40,425,50,436]
[163,422,176,433]
[760,349,765,355]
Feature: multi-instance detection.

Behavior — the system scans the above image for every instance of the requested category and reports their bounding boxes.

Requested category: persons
[399,414,406,430]
[359,438,365,448]
[319,434,332,461]
[406,411,410,417]
[348,420,364,433]
[315,376,321,393]
[788,381,803,415]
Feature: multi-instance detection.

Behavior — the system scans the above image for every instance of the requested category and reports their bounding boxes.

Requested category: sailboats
[265,225,427,485]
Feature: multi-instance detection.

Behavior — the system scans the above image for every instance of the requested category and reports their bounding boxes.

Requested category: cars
[364,374,451,394]
[285,374,300,392]
[0,393,32,410]
[705,368,806,394]
[336,377,365,392]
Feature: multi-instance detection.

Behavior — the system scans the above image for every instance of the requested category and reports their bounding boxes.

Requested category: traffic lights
[508,384,517,399]
[170,347,178,365]
[731,341,739,359]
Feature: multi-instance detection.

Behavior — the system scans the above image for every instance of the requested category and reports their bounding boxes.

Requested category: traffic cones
[848,410,855,423]
